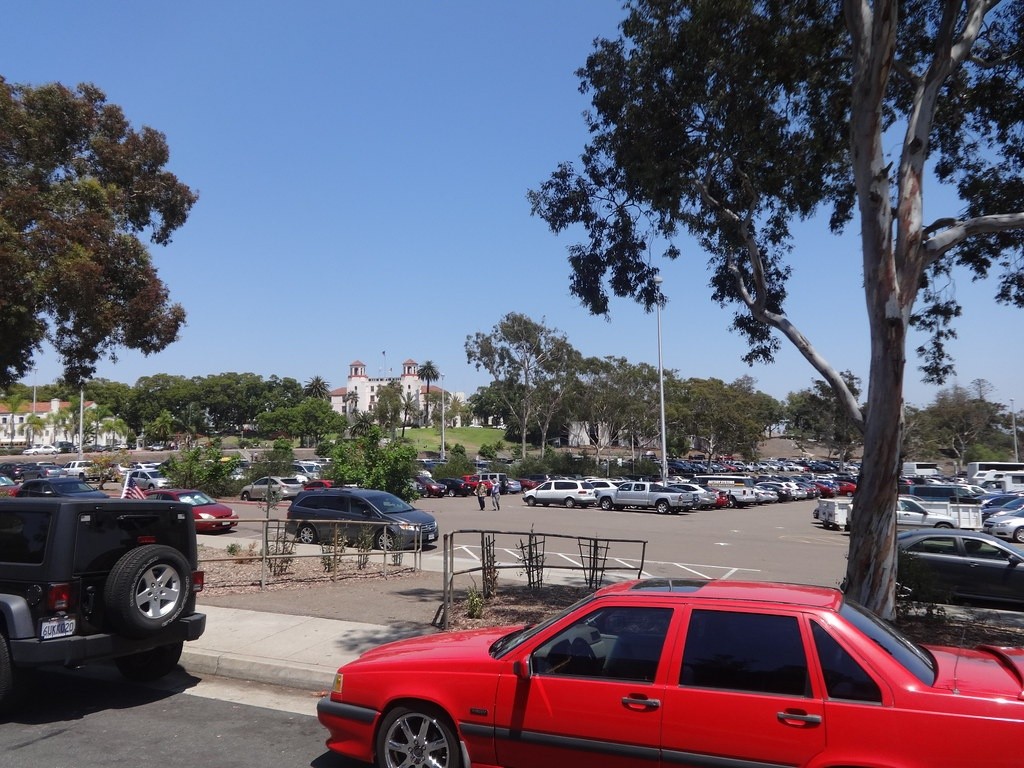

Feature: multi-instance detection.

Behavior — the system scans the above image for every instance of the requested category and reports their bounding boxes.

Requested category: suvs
[0,494,207,724]
[845,494,958,531]
[15,476,110,498]
[285,486,439,551]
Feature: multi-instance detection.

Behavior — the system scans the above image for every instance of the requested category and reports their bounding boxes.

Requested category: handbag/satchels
[481,492,487,497]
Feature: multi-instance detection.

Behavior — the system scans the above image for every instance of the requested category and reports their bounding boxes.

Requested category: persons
[489,477,501,511]
[474,479,487,511]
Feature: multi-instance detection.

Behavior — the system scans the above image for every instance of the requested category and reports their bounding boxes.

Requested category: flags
[122,476,145,500]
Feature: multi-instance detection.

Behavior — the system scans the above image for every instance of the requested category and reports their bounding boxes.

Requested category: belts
[492,493,498,494]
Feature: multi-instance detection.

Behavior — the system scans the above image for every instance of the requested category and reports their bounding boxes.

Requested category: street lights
[439,372,447,459]
[31,367,39,444]
[1009,398,1019,463]
[650,275,668,487]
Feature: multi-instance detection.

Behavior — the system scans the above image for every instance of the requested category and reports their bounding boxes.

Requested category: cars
[142,488,239,534]
[0,472,20,497]
[894,527,1024,613]
[129,469,173,491]
[151,442,164,451]
[239,475,305,502]
[23,440,129,455]
[313,572,1024,768]
[0,460,163,482]
[415,452,1024,543]
[228,456,333,481]
[305,480,333,490]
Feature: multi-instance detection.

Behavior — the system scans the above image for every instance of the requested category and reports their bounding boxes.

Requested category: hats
[478,479,482,483]
